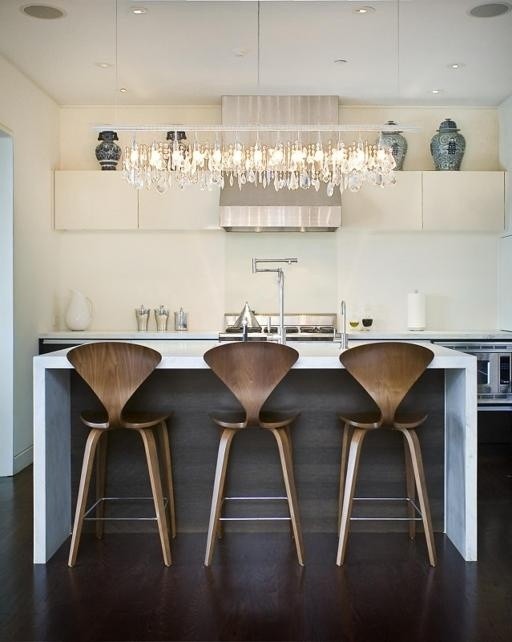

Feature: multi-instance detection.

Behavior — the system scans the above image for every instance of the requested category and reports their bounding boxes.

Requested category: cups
[152,304,169,331]
[347,314,362,329]
[362,313,375,328]
[134,305,151,332]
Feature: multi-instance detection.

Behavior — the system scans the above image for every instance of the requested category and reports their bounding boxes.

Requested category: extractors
[219,94,344,234]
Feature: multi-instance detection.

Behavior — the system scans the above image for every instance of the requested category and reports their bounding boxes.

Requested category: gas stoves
[220,313,336,340]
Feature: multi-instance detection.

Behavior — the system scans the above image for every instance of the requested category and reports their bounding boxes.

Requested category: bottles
[173,306,189,332]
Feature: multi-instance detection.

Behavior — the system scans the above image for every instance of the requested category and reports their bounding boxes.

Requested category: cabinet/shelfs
[138,170,223,232]
[341,170,423,230]
[424,170,505,230]
[55,171,138,233]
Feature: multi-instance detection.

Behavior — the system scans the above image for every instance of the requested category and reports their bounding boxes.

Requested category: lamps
[93,125,406,198]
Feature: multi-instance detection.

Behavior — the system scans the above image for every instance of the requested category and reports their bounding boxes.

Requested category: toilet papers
[407,293,427,331]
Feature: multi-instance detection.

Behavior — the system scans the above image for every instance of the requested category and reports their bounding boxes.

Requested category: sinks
[292,342,362,357]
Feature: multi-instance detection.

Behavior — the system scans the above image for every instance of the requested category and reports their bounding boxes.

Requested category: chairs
[65,342,179,568]
[202,341,308,568]
[334,342,439,568]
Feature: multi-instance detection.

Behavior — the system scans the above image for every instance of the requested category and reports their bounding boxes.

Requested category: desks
[31,337,480,567]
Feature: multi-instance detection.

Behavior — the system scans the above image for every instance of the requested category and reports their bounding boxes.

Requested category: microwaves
[438,342,512,405]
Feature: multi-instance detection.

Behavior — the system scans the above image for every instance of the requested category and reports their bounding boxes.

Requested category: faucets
[276,269,287,346]
[340,300,349,349]
